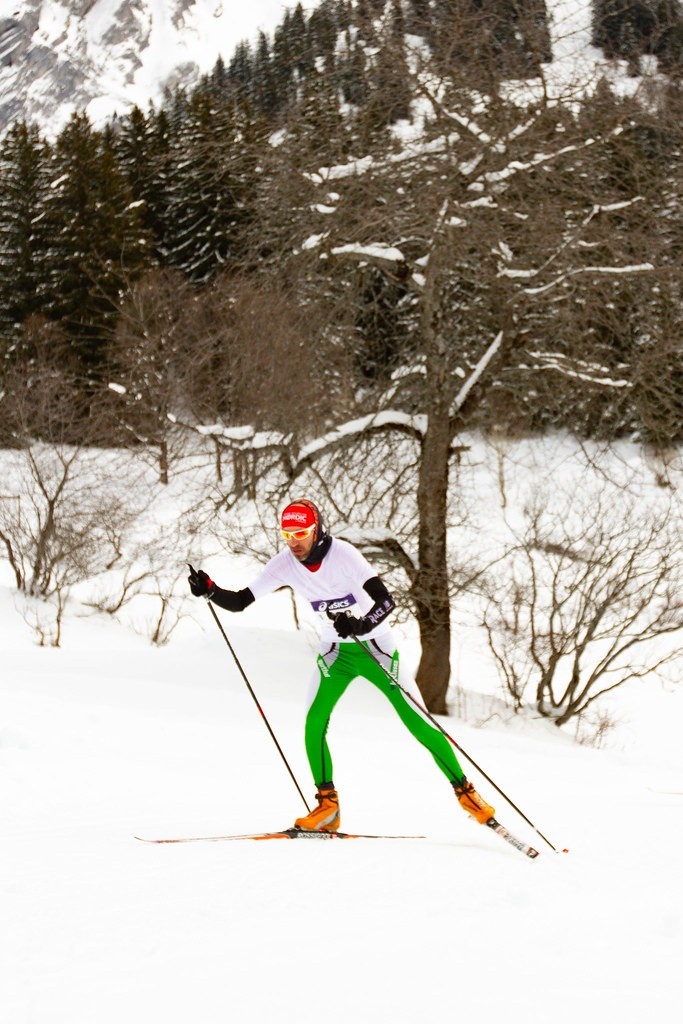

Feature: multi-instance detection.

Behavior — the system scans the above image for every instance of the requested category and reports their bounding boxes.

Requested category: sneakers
[295,782,340,829]
[451,775,495,824]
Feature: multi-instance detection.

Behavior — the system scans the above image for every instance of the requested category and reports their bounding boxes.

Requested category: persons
[187,500,496,831]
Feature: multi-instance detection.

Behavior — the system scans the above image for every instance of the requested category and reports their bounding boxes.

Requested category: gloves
[334,610,367,639]
[188,570,216,599]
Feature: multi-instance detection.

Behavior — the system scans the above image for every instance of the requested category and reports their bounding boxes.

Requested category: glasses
[282,523,316,540]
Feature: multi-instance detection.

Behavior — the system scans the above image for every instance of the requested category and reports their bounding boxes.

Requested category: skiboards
[131,812,541,860]
